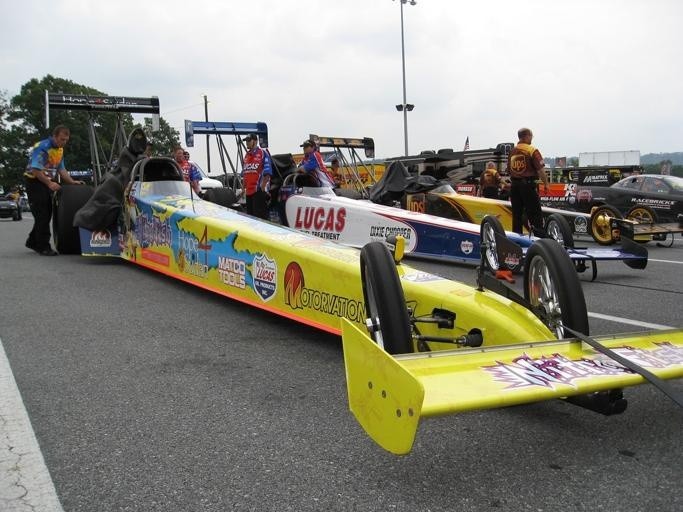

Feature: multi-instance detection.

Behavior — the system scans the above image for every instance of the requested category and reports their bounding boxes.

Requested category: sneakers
[23,240,58,257]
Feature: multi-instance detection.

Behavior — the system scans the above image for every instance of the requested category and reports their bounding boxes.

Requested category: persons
[296,141,336,191]
[480,162,507,199]
[25,125,86,256]
[171,147,203,195]
[328,158,346,189]
[3,184,26,221]
[506,128,550,238]
[145,142,153,159]
[240,133,273,221]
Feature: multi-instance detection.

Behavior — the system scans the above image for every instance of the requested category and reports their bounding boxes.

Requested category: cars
[0,192,31,222]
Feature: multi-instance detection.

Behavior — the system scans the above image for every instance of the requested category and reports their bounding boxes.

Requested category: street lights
[392,0,420,157]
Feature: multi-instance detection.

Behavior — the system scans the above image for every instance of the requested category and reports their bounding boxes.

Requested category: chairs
[204,187,243,212]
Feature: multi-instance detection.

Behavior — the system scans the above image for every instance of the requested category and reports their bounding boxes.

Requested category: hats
[299,139,316,149]
[241,132,258,142]
